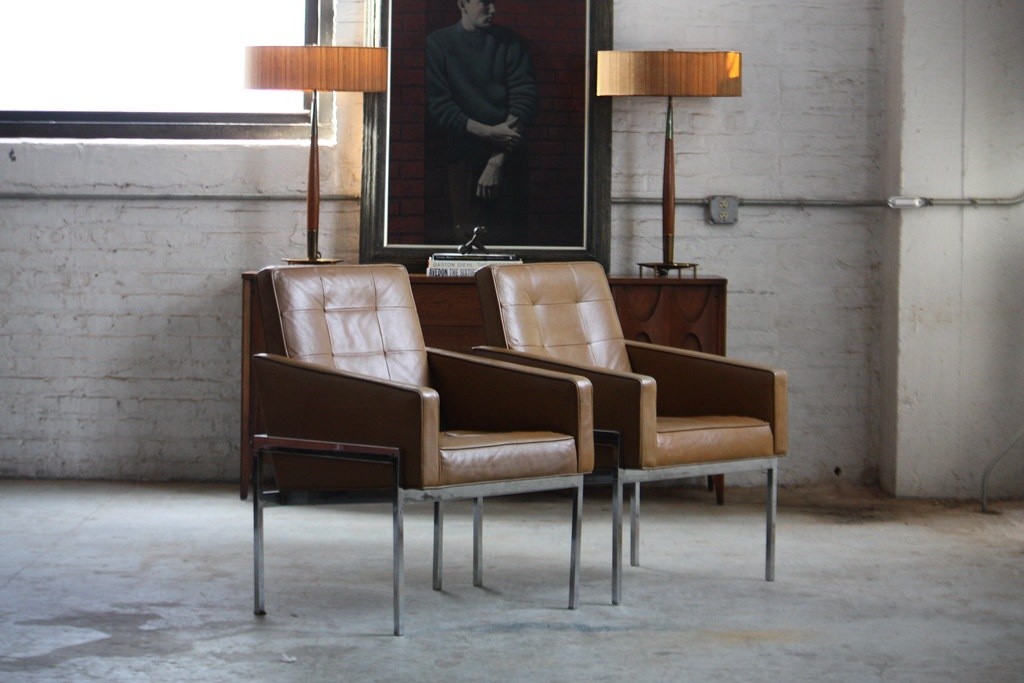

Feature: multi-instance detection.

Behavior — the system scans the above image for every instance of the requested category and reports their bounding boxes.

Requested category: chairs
[252,264,594,636]
[471,261,788,606]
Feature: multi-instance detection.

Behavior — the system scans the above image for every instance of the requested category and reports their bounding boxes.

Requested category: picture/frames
[359,0,613,274]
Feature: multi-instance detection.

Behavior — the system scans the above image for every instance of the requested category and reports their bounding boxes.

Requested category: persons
[423,0,541,244]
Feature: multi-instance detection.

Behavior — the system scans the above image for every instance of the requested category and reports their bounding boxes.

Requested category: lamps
[244,42,390,265]
[596,49,742,278]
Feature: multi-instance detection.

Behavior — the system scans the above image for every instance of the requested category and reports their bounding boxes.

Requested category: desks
[238,271,728,507]
